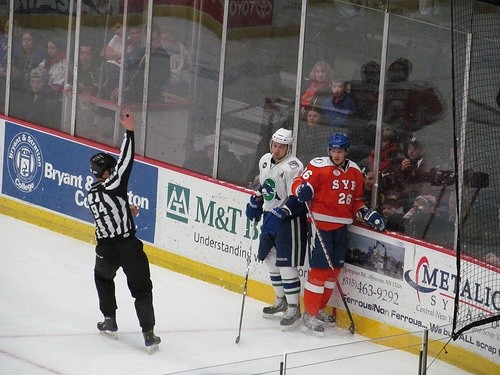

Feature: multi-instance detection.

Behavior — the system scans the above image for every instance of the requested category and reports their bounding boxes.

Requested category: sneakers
[97,314,119,340]
[262,296,288,318]
[318,311,336,329]
[301,312,325,338]
[280,306,300,331]
[143,330,161,354]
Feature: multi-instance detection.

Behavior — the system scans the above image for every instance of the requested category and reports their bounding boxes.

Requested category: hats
[408,136,423,148]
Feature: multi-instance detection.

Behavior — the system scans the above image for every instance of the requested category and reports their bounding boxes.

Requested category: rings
[126,114,129,117]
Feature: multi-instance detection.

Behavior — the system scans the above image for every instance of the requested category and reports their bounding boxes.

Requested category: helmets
[270,127,294,155]
[327,132,351,155]
[89,154,119,176]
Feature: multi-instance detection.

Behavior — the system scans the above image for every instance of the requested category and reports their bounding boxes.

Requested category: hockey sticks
[304,201,355,334]
[235,218,256,344]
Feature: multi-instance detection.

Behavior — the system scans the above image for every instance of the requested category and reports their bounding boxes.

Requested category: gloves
[261,208,287,235]
[362,209,385,231]
[245,194,264,222]
[297,184,312,203]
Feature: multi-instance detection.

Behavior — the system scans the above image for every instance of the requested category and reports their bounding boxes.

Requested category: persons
[0,15,442,232]
[292,133,385,332]
[88,107,161,346]
[245,128,304,326]
